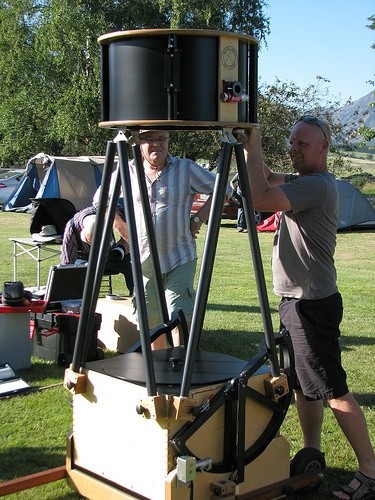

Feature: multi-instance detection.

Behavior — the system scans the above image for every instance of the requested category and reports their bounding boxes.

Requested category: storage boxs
[0,304,31,371]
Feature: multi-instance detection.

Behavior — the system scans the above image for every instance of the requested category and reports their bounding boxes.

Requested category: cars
[191,193,263,227]
[0,168,26,205]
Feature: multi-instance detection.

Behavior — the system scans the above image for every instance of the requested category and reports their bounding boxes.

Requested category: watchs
[190,215,204,227]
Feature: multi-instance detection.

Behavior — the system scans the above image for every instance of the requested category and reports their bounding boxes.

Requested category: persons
[230,115,375,500]
[93,129,233,350]
[61,206,134,297]
[231,173,248,233]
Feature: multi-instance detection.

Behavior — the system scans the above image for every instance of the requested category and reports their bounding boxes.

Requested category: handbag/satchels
[228,190,243,209]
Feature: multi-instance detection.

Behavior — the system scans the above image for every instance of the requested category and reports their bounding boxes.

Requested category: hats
[32,225,61,242]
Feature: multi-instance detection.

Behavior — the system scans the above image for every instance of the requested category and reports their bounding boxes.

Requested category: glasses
[293,115,327,140]
[140,137,169,144]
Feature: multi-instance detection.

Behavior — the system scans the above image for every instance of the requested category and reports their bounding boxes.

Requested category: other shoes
[238,227,247,233]
[330,470,375,500]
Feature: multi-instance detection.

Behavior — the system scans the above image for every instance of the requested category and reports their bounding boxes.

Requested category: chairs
[9,197,76,291]
[62,217,119,299]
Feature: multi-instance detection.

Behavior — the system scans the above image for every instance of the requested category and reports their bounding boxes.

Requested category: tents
[2,153,132,214]
[255,179,375,232]
